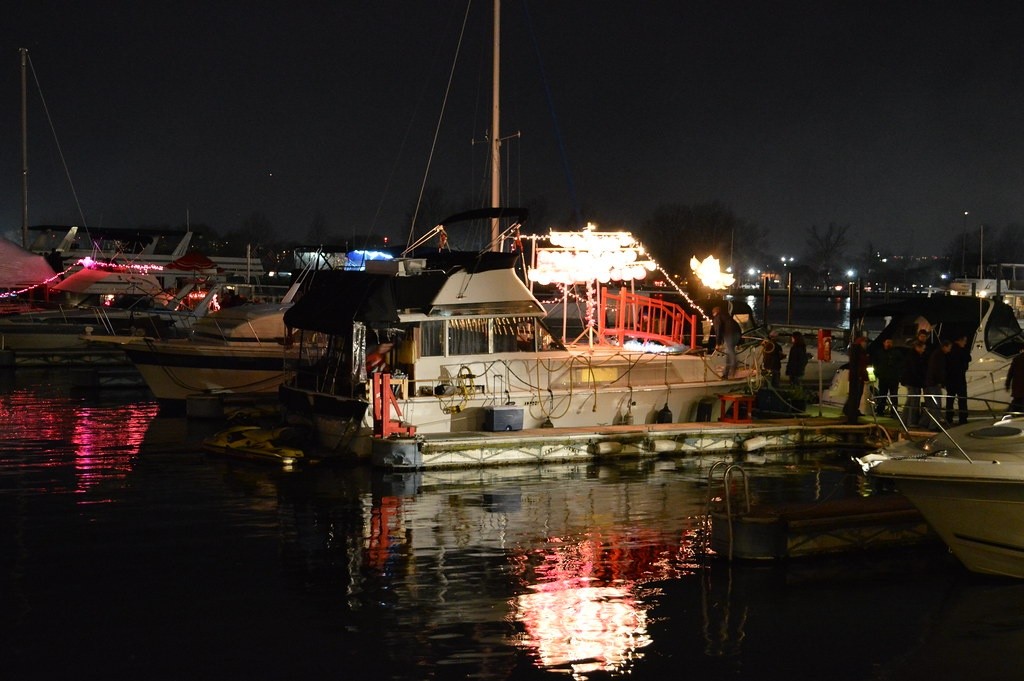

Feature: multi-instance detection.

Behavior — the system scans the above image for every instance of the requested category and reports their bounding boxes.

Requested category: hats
[918,329,927,336]
[770,331,778,336]
[855,336,866,343]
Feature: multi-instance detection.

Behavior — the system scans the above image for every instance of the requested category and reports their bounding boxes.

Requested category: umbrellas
[164,250,218,278]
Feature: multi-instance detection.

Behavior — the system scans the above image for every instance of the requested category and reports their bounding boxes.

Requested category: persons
[1005,352,1024,412]
[712,307,742,377]
[872,329,971,431]
[785,331,808,412]
[845,336,869,422]
[366,341,394,370]
[763,330,787,388]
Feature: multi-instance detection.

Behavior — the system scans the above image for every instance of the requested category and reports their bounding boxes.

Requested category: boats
[0,0,1024,590]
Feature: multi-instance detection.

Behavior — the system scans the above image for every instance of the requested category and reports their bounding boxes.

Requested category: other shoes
[718,376,728,380]
[855,411,865,416]
[958,418,969,424]
[901,422,920,428]
[930,427,943,432]
